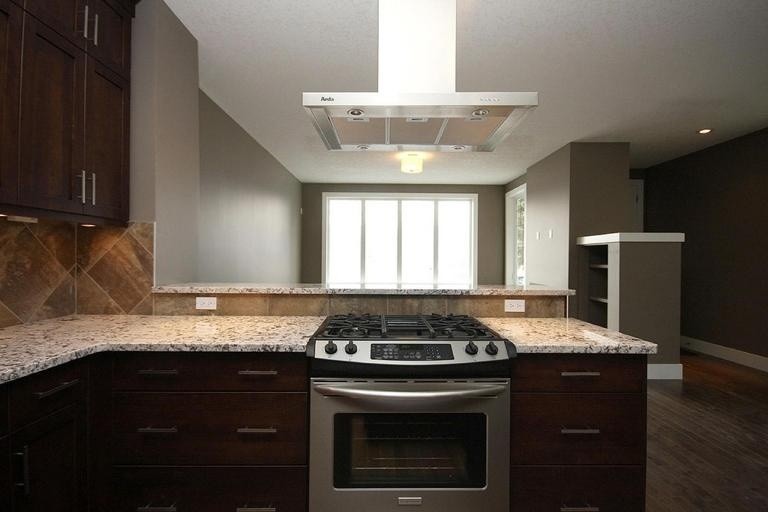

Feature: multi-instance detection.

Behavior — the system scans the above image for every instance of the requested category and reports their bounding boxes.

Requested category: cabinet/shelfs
[1,350,100,512]
[521,140,628,323]
[100,345,307,512]
[0,1,135,230]
[577,232,685,381]
[511,351,649,511]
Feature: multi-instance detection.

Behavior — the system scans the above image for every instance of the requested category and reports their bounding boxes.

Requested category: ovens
[309,380,508,511]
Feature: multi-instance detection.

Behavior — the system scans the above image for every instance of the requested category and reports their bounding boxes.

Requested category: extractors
[298,0,535,154]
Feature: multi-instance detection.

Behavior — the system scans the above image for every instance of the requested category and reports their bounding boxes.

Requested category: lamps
[400,152,423,174]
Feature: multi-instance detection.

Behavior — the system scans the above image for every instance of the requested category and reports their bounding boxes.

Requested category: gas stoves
[304,312,514,373]
